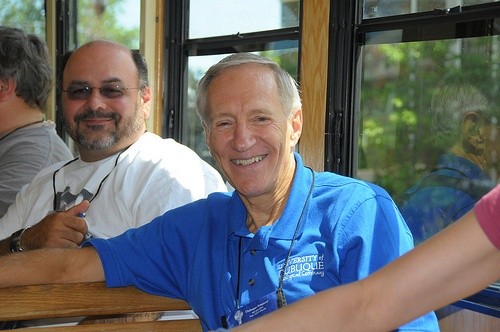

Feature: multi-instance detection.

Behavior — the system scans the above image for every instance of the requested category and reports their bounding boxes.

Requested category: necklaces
[1,118,45,141]
[52,147,130,218]
[220,165,315,331]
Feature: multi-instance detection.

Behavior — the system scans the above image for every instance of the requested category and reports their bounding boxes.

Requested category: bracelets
[9,227,30,253]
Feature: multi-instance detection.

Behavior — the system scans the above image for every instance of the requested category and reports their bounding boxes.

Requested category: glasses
[60,84,142,100]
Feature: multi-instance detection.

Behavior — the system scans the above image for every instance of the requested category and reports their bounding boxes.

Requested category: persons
[0,25,75,220]
[0,51,441,332]
[397,69,499,247]
[1,39,227,256]
[226,183,500,332]
